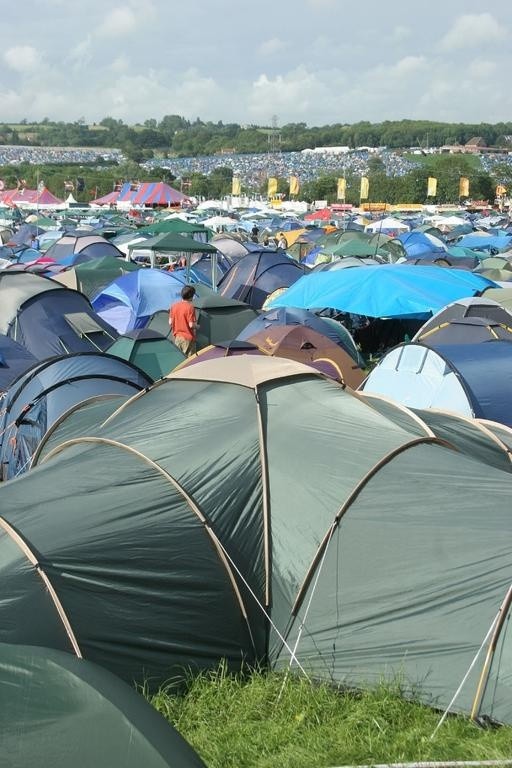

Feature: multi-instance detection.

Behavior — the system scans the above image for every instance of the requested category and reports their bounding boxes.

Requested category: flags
[232,176,242,195]
[360,175,369,198]
[459,176,470,196]
[290,175,300,195]
[267,176,277,198]
[336,177,347,200]
[427,177,437,196]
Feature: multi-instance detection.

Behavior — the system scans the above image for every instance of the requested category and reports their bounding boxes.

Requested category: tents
[0,209,512,763]
